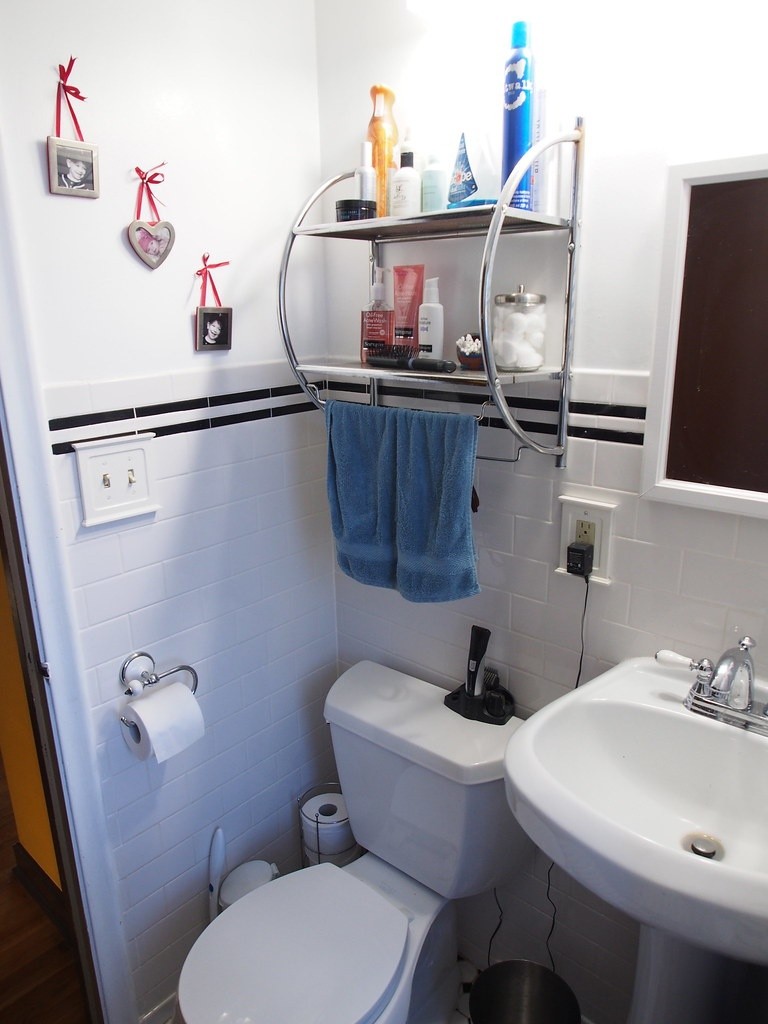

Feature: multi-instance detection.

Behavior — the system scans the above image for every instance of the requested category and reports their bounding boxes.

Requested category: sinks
[529,696,767,877]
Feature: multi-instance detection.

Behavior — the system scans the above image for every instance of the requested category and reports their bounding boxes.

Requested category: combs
[366,343,457,374]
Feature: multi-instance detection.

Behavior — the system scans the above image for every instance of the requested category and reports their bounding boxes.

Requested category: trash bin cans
[467,959,580,1024]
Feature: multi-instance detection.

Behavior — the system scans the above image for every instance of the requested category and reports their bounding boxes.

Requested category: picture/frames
[47,135,99,198]
[196,305,233,351]
[129,220,175,270]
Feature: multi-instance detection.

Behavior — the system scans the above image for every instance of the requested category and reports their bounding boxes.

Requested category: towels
[324,399,480,604]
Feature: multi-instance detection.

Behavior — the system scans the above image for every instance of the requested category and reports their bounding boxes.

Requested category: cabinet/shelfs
[277,117,580,466]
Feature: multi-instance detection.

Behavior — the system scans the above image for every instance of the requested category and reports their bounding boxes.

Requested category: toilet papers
[299,792,364,867]
[118,683,207,763]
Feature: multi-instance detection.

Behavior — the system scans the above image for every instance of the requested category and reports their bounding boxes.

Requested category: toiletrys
[420,152,448,215]
[359,265,443,363]
[356,85,420,215]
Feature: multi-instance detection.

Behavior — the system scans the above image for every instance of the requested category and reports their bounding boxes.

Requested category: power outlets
[556,495,615,586]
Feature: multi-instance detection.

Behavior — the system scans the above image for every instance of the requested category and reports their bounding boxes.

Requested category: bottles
[492,294,546,372]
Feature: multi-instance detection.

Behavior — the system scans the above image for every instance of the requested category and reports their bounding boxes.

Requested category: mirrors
[645,168,767,517]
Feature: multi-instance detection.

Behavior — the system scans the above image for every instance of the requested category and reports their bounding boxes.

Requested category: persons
[58,157,92,190]
[137,229,169,257]
[203,315,223,346]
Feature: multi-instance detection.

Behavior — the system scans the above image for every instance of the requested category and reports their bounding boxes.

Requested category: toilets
[175,657,536,1024]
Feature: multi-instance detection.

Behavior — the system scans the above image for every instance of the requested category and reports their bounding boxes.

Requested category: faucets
[707,636,755,711]
